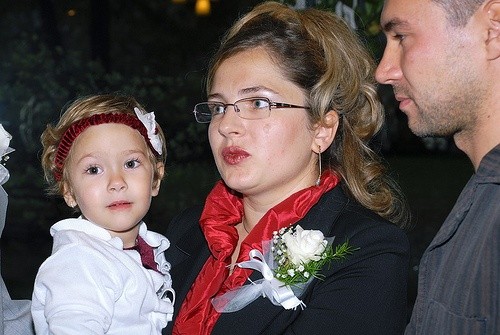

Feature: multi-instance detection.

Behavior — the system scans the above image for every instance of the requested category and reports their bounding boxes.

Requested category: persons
[376,0,500,335]
[162,1,415,335]
[30,94,176,335]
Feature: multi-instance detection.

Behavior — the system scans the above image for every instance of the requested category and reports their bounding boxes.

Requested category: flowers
[269,225,361,285]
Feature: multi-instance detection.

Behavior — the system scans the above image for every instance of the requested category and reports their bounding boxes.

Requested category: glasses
[194,97,312,124]
